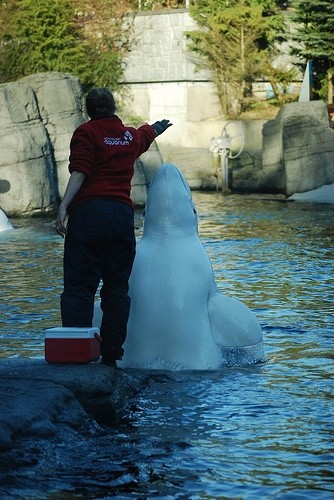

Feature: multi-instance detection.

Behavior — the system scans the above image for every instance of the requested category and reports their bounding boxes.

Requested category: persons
[51,86,174,367]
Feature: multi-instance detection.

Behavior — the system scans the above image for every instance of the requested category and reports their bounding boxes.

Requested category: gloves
[151,119,172,136]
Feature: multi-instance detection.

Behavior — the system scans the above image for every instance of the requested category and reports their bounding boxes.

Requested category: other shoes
[99,358,118,369]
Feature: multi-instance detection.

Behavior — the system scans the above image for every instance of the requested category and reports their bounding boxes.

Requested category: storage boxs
[45,327,101,362]
